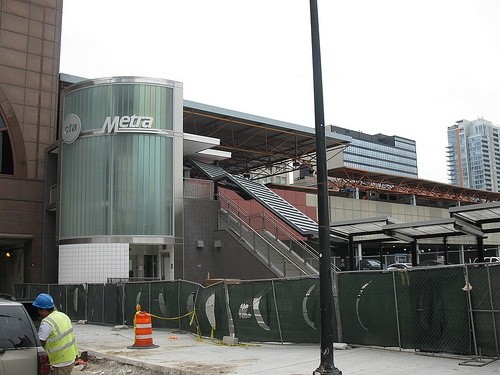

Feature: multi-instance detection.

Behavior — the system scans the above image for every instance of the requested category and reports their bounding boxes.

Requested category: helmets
[32,293,54,309]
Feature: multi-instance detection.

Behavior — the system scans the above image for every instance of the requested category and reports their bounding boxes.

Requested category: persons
[32,293,79,375]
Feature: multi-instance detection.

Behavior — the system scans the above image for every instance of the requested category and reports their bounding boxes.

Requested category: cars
[472,256,500,262]
[388,262,412,269]
[361,258,384,271]
[1,290,51,375]
